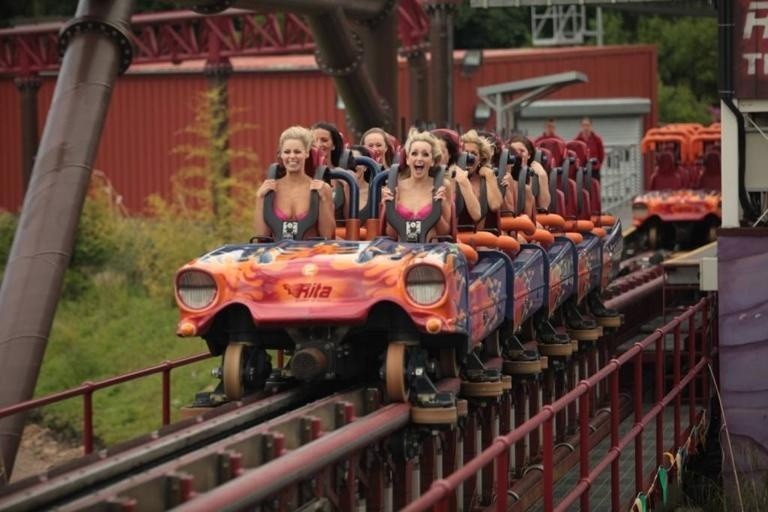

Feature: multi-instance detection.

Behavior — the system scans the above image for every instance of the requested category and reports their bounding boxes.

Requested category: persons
[428,132,481,221]
[253,125,336,244]
[478,133,518,213]
[573,117,605,184]
[360,127,394,171]
[311,121,350,219]
[460,130,504,212]
[349,145,371,211]
[508,135,550,213]
[525,169,535,221]
[535,119,564,145]
[381,134,451,242]
